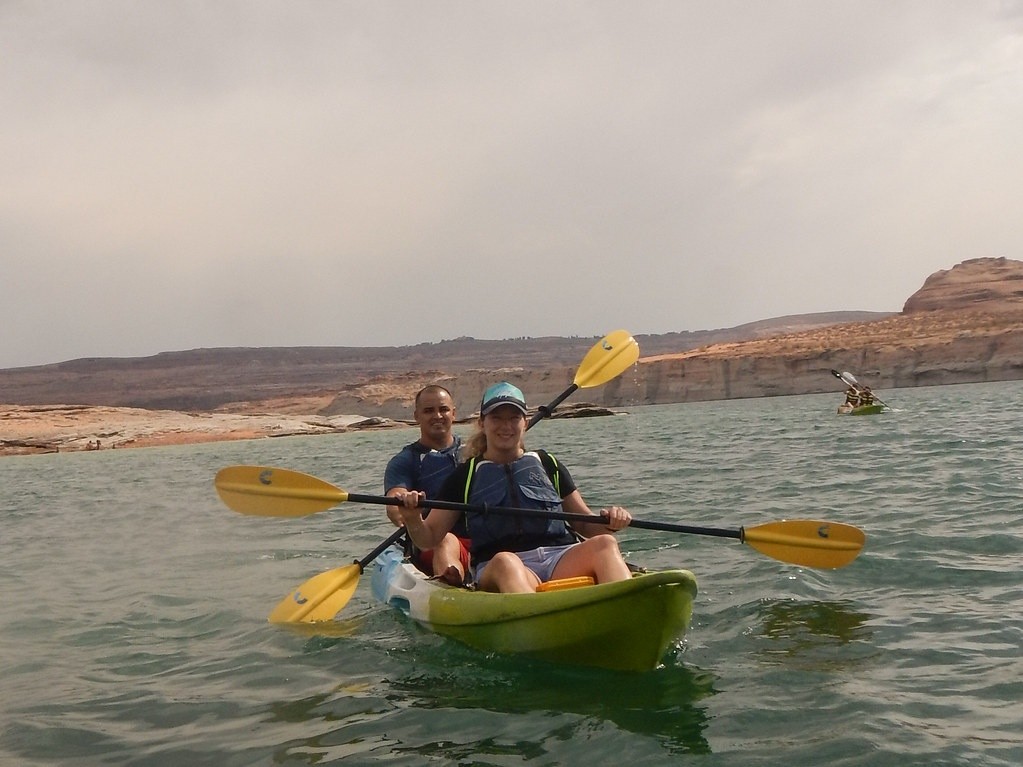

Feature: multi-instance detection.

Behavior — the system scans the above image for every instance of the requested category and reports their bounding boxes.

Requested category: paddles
[831,369,859,393]
[214,465,866,571]
[268,329,640,623]
[843,372,893,411]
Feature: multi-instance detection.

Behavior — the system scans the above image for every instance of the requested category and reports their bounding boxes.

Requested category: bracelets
[605,526,619,532]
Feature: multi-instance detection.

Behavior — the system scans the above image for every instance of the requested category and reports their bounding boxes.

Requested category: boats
[836,403,886,415]
[369,533,699,674]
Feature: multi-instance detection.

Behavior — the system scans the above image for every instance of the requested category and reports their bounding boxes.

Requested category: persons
[383,385,475,584]
[395,382,633,594]
[843,383,879,411]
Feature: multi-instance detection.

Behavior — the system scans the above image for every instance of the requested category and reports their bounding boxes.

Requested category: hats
[481,381,529,415]
